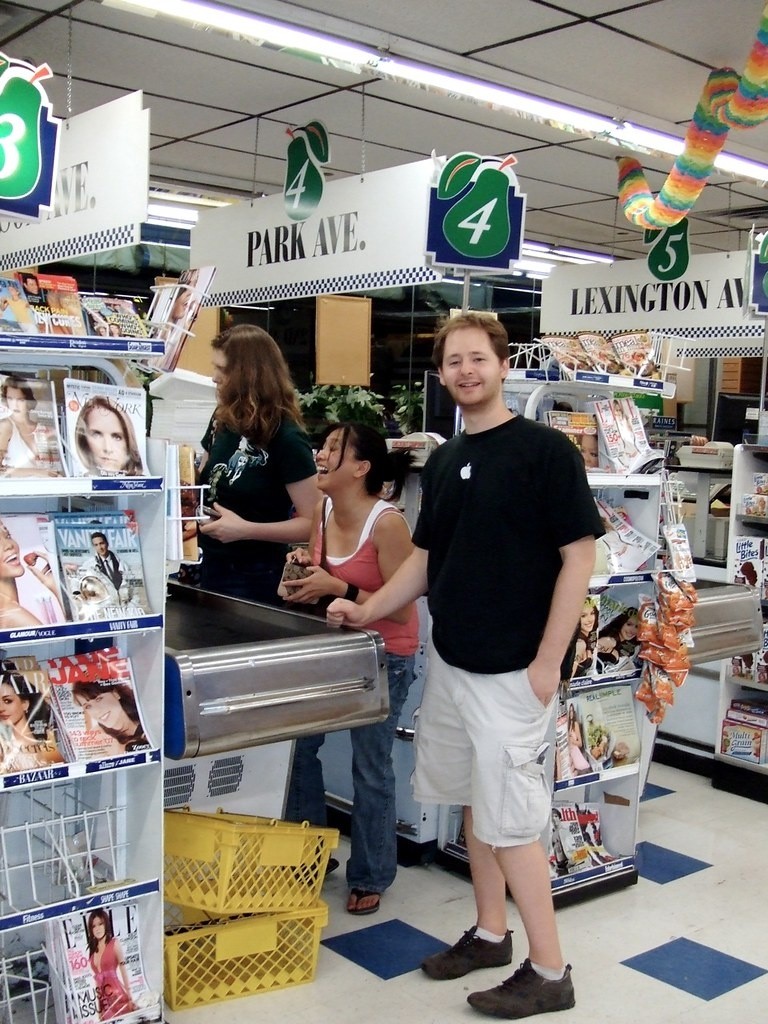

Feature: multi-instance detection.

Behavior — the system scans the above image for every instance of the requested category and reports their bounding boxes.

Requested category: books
[43,899,161,1024]
[540,394,666,878]
[0,265,216,788]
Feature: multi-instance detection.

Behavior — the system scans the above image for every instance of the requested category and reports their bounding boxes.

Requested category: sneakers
[421,925,515,980]
[467,958,575,1019]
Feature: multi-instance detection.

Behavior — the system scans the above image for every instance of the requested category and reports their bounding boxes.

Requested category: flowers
[294,380,424,438]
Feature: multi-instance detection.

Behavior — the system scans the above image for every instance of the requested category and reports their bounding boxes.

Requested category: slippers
[296,858,339,886]
[347,888,381,915]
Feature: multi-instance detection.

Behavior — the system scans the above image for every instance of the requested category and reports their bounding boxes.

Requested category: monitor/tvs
[713,392,768,447]
[422,370,458,441]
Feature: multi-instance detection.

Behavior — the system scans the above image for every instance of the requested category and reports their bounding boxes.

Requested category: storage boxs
[163,805,340,914]
[719,471,768,765]
[164,900,330,1009]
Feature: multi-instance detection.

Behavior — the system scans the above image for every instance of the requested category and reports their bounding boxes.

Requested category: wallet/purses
[277,555,320,604]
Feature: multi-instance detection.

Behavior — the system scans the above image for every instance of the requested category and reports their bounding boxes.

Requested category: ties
[105,560,113,577]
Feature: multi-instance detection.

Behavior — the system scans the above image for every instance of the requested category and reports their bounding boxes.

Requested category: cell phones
[199,505,220,518]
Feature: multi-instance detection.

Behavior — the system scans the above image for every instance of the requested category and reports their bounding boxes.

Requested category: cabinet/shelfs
[0,332,170,1024]
[712,442,768,805]
[433,332,698,912]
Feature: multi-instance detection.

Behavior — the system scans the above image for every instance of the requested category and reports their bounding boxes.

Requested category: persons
[0,375,64,477]
[0,671,65,774]
[327,312,605,1021]
[596,607,640,672]
[85,908,139,1020]
[69,672,151,764]
[81,532,141,606]
[74,395,144,477]
[160,269,199,341]
[0,283,40,333]
[109,323,122,337]
[549,807,579,875]
[283,419,422,917]
[560,595,601,681]
[193,324,318,612]
[95,326,107,338]
[0,521,67,628]
[567,702,589,775]
[22,274,71,335]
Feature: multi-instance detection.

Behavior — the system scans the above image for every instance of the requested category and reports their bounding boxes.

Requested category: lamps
[99,0,768,279]
